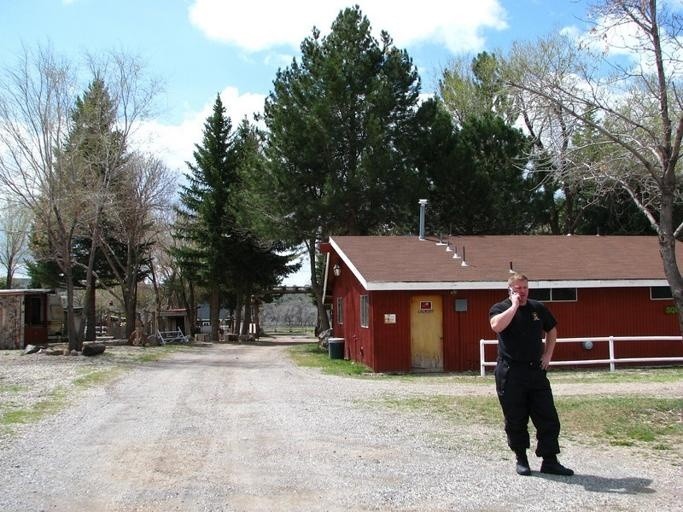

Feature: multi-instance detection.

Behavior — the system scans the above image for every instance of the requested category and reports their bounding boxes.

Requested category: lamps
[332,258,342,277]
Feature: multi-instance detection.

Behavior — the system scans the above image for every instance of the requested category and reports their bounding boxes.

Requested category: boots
[540,454,573,476]
[516,453,531,476]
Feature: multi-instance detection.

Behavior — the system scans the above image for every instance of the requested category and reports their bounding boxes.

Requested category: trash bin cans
[328,338,344,359]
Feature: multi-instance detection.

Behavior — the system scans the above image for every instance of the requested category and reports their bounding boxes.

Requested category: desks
[223,334,238,341]
[194,334,209,342]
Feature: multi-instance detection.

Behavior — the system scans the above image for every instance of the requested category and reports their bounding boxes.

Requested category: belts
[498,358,540,368]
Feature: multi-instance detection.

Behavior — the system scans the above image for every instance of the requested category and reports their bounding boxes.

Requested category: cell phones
[507,285,514,293]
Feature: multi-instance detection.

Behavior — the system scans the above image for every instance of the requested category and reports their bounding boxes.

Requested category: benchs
[46,320,64,342]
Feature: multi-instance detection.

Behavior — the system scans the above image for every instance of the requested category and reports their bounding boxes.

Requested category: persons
[109,299,116,316]
[488,273,575,476]
